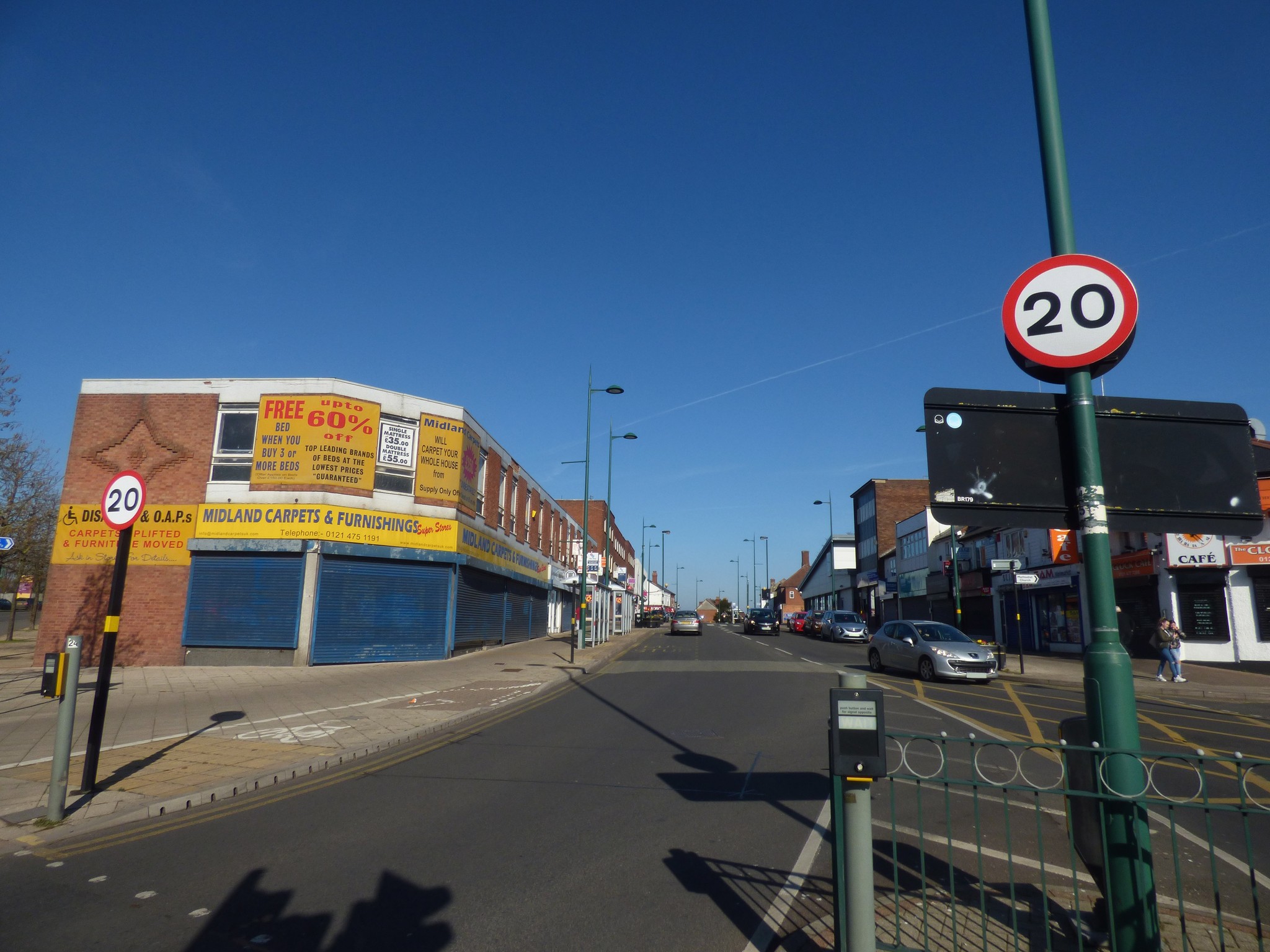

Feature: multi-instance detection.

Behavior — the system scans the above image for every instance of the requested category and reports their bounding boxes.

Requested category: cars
[650,610,673,623]
[867,619,999,685]
[0,598,11,610]
[743,608,781,636]
[37,601,42,611]
[671,610,703,636]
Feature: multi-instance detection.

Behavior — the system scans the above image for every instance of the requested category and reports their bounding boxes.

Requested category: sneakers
[1174,675,1186,682]
[1155,674,1166,682]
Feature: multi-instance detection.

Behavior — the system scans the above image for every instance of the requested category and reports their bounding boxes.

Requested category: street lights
[696,580,703,612]
[730,560,739,619]
[719,591,725,623]
[661,530,671,608]
[575,385,625,649]
[603,432,638,582]
[740,576,749,610]
[677,567,684,610]
[744,539,757,608]
[761,537,769,589]
[641,525,657,617]
[814,501,837,611]
[648,545,660,614]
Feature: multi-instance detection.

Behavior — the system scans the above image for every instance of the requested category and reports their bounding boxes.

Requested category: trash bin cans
[635,613,642,628]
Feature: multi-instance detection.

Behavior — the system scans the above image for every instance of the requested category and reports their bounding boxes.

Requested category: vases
[642,618,665,628]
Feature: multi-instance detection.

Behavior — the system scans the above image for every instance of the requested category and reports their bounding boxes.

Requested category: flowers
[647,614,662,618]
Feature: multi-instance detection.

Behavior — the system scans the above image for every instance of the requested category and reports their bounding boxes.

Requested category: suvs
[15,597,35,610]
[804,610,828,639]
[821,610,871,642]
[789,611,806,634]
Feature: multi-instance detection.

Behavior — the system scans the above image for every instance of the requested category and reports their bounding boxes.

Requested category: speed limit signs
[101,470,147,530]
[1001,254,1139,369]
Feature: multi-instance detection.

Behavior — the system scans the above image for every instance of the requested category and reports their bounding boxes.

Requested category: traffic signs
[1015,574,1039,584]
[0,537,15,550]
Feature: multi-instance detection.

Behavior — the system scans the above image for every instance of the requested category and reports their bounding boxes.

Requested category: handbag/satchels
[1149,633,1157,648]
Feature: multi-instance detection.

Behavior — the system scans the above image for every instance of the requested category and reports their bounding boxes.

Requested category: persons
[575,607,580,638]
[1155,618,1186,683]
[616,603,622,630]
[908,627,929,640]
[721,610,728,621]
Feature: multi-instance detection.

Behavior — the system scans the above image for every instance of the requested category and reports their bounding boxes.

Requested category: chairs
[760,612,764,615]
[753,612,758,616]
[678,614,683,617]
[691,614,695,617]
